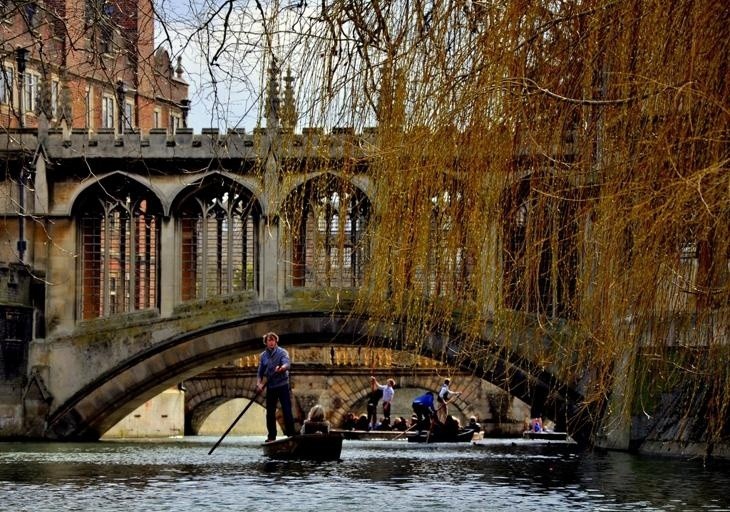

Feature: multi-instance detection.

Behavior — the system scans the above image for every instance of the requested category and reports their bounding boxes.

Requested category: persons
[534,423,541,433]
[256,332,299,442]
[301,405,330,435]
[465,417,481,432]
[343,377,462,443]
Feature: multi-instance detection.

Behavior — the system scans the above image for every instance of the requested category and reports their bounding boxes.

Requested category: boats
[261,433,342,460]
[332,424,486,442]
[523,426,570,441]
[404,428,475,444]
